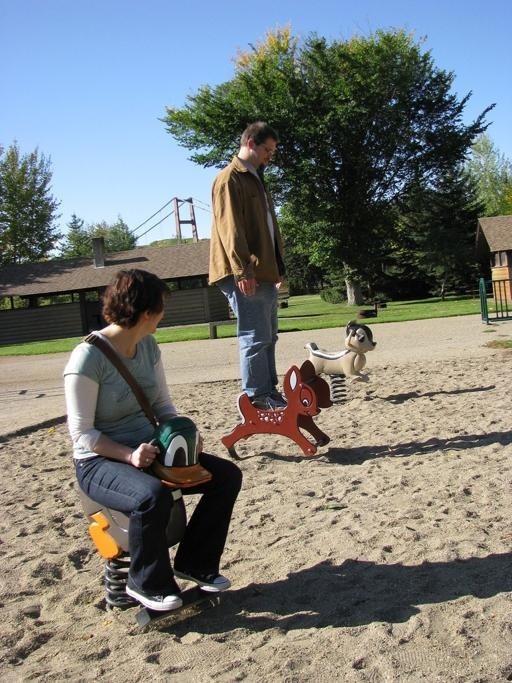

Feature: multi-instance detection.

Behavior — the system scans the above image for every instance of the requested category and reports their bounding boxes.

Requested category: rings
[145,458,149,463]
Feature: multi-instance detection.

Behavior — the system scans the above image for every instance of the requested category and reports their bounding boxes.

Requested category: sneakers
[250,387,287,409]
[173,566,232,595]
[123,584,183,612]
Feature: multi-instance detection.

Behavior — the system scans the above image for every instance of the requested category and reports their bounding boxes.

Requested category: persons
[62,270,243,611]
[206,123,289,409]
